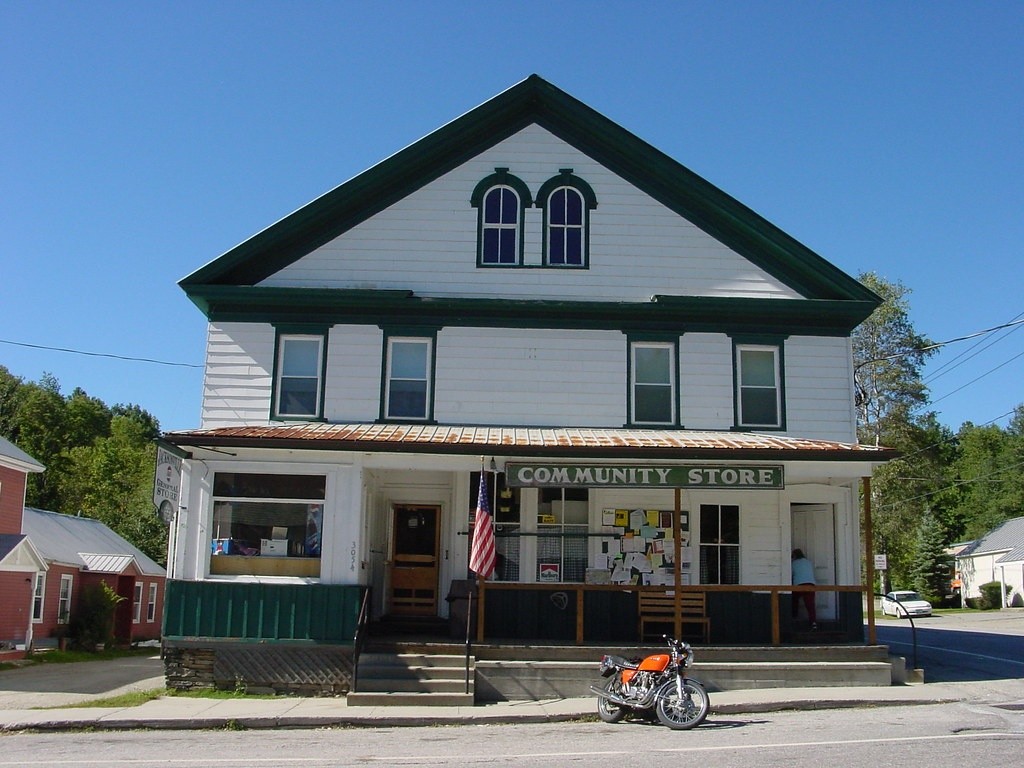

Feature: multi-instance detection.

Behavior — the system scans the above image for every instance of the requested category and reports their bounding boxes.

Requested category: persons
[790,548,818,632]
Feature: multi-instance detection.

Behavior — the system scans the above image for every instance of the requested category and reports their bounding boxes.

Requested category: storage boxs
[212,538,235,555]
[260,539,289,557]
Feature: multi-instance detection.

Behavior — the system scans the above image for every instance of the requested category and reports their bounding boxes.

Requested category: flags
[470,455,495,580]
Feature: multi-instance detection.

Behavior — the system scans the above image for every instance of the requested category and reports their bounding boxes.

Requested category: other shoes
[808,623,817,633]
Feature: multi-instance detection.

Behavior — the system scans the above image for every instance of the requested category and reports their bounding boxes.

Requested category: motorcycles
[589,634,709,730]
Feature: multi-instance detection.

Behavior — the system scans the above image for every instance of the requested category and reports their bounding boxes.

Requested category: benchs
[636,591,712,645]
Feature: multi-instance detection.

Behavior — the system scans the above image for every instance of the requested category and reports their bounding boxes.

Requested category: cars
[879,590,932,619]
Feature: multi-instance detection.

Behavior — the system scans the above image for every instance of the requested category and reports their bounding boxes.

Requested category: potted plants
[72,578,129,652]
